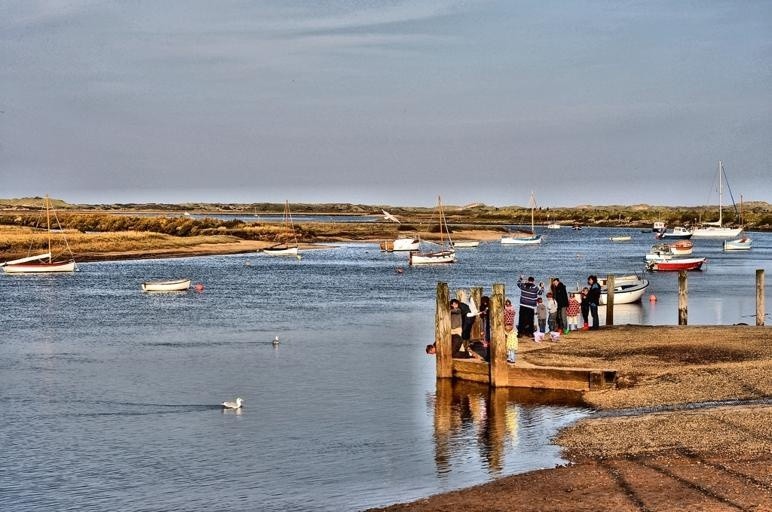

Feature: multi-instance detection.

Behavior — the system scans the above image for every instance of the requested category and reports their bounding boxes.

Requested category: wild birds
[273,336,279,344]
[382,209,400,224]
[221,398,244,409]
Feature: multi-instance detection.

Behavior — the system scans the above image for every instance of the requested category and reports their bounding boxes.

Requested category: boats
[141,278,192,292]
[547,212,692,243]
[449,240,481,248]
[651,239,693,257]
[567,274,650,306]
[379,237,420,251]
[641,257,706,272]
[184,210,190,215]
[1,253,51,269]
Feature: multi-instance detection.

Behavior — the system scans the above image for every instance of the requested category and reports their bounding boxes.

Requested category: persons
[426,274,602,363]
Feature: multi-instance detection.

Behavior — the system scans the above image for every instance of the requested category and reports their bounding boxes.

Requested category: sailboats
[2,194,76,272]
[685,159,743,238]
[409,195,456,267]
[261,198,299,258]
[724,195,753,251]
[500,192,542,245]
[254,206,258,217]
